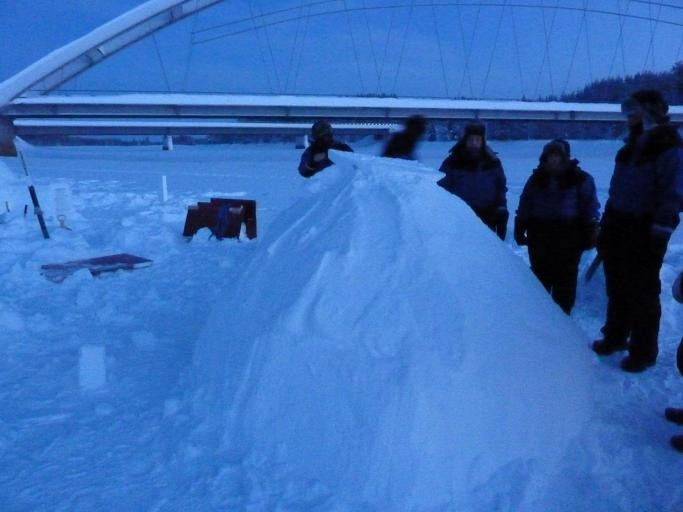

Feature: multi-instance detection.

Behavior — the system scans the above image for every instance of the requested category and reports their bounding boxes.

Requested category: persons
[437,122,509,240]
[297,118,354,178]
[514,136,602,315]
[664,270,682,451]
[381,112,426,160]
[589,87,682,374]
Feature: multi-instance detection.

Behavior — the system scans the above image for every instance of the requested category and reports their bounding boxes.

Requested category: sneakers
[592,336,658,371]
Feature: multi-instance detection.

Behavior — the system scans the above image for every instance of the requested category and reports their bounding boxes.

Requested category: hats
[623,89,670,125]
[539,140,570,168]
[312,122,333,137]
[449,124,484,151]
